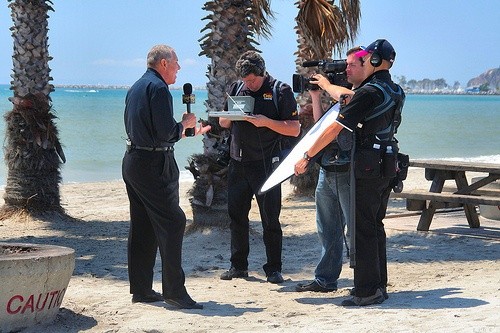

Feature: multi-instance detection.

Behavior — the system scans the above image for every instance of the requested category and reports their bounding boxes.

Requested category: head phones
[370,39,385,67]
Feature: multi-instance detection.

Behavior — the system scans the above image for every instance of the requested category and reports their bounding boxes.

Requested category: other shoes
[342,289,388,306]
[295,281,337,292]
[221,266,248,280]
[132,289,163,302]
[164,295,203,309]
[267,271,283,282]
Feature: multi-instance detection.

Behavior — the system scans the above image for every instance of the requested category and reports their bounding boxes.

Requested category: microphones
[182,83,196,136]
[302,61,325,67]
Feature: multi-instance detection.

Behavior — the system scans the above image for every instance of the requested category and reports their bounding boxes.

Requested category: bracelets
[304,151,310,161]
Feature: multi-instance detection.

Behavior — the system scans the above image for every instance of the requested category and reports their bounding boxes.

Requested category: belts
[321,164,349,172]
[131,144,174,151]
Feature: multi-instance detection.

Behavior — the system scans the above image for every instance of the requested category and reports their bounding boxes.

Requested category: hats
[354,38,396,61]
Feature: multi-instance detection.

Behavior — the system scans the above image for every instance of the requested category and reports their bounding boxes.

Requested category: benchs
[471,188,500,196]
[389,191,500,211]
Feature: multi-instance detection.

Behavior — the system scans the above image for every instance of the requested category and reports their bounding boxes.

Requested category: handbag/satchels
[256,80,302,178]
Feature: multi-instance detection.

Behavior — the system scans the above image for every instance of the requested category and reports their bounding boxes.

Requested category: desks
[408,159,500,232]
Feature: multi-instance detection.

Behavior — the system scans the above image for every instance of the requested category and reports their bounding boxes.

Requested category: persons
[218,51,301,283]
[121,44,204,311]
[294,39,405,306]
[295,45,365,293]
